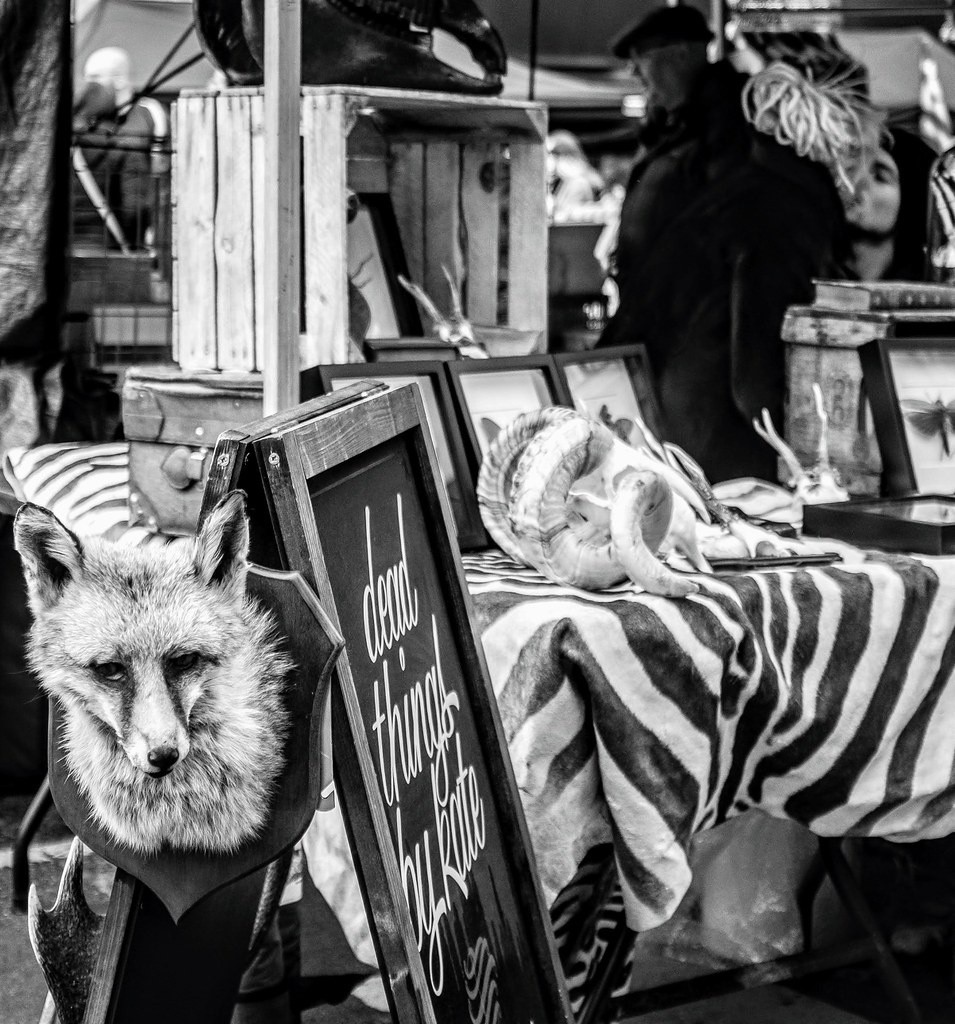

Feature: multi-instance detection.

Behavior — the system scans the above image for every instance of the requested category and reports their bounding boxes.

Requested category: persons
[68,46,170,251]
[842,130,940,282]
[592,7,861,487]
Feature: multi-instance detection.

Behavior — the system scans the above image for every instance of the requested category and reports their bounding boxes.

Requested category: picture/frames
[443,354,570,550]
[301,360,487,552]
[552,343,665,445]
[802,494,955,556]
[364,337,463,362]
[347,191,424,354]
[804,338,955,499]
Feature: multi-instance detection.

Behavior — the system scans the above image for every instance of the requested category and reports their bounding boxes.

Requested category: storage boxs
[122,365,261,536]
[65,247,169,347]
[170,84,550,385]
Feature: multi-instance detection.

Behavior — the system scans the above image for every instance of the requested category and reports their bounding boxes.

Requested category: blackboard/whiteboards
[247,383,575,1024]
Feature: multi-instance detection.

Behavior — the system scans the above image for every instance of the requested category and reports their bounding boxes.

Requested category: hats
[612,3,715,59]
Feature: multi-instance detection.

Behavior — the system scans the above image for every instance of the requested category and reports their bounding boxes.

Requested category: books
[781,279,955,349]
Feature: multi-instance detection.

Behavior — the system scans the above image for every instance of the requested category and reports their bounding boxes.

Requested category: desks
[461,533,955,1024]
